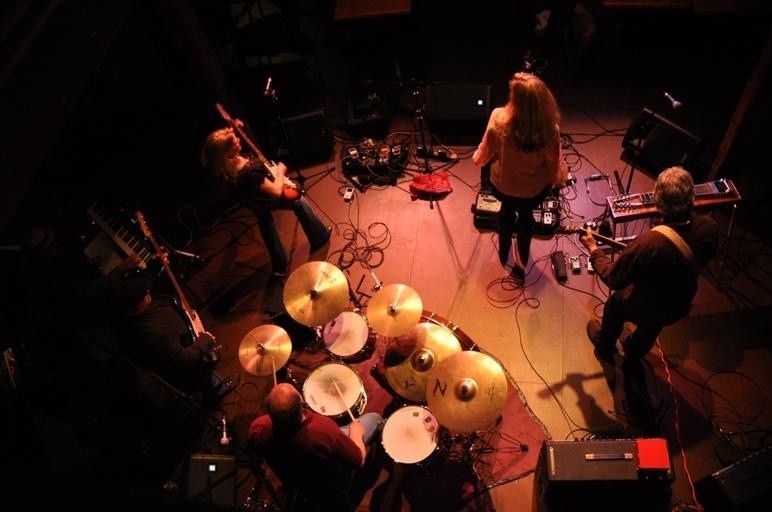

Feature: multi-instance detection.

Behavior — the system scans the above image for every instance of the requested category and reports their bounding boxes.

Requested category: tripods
[271,97,335,198]
[404,79,457,209]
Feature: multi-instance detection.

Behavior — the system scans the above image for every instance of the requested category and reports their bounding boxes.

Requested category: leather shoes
[618,324,636,347]
[587,318,616,366]
[312,224,333,250]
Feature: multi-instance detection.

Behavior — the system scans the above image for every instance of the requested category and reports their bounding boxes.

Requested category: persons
[471,72,562,279]
[200,118,333,271]
[246,381,386,497]
[579,167,721,363]
[23,217,214,297]
[106,273,222,402]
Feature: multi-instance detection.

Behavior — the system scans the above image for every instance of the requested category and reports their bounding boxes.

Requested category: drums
[301,361,368,426]
[382,405,456,464]
[321,307,376,363]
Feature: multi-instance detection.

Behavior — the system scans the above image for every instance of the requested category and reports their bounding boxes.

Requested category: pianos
[88,201,165,277]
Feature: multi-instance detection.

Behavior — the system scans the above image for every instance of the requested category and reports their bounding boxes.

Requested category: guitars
[134,209,221,366]
[215,102,303,203]
[553,225,628,251]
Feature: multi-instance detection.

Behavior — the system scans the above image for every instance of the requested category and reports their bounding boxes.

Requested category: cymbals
[426,351,508,433]
[238,324,292,376]
[366,283,423,337]
[383,322,463,402]
[283,261,349,326]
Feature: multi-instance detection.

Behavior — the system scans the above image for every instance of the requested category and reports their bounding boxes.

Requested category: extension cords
[587,256,595,272]
[571,256,581,270]
[344,187,355,200]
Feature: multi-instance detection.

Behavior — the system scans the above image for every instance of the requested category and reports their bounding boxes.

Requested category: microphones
[664,93,683,111]
[524,58,531,70]
[615,201,644,208]
[220,415,229,446]
[264,77,274,97]
